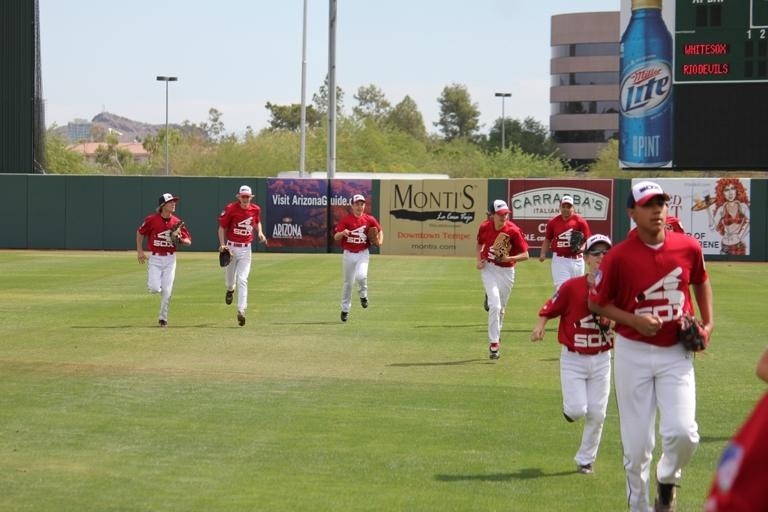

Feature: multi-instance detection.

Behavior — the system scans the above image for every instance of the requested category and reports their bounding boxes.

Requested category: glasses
[590,249,609,256]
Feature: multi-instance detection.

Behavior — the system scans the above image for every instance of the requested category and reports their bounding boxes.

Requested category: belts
[557,253,583,258]
[567,346,599,354]
[488,259,511,266]
[152,251,173,255]
[350,250,359,253]
[228,241,249,246]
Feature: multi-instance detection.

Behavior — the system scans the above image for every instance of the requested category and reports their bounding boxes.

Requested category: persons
[587,182,716,512]
[216,185,268,326]
[136,193,192,326]
[700,345,768,512]
[706,178,750,255]
[530,235,615,474]
[331,194,384,322]
[540,196,592,296]
[475,199,530,359]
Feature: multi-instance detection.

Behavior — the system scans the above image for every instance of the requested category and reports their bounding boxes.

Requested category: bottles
[619,0,674,169]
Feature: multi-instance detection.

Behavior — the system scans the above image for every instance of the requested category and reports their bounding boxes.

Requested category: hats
[584,234,613,250]
[159,192,179,206]
[352,194,366,203]
[489,199,511,216]
[627,181,670,207]
[235,184,255,201]
[560,195,573,206]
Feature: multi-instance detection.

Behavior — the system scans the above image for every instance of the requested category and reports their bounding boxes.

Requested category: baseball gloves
[571,231,584,252]
[219,248,231,266]
[680,317,710,350]
[368,227,380,247]
[171,218,185,252]
[494,232,512,262]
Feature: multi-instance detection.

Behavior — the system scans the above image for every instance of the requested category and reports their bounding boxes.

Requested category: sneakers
[159,319,167,326]
[341,311,349,321]
[579,463,593,475]
[654,477,678,512]
[237,313,245,326]
[563,413,573,424]
[361,297,369,308]
[226,290,234,304]
[484,294,489,311]
[490,343,500,359]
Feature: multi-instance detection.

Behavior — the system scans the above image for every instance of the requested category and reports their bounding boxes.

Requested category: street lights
[495,92,511,151]
[156,76,178,177]
